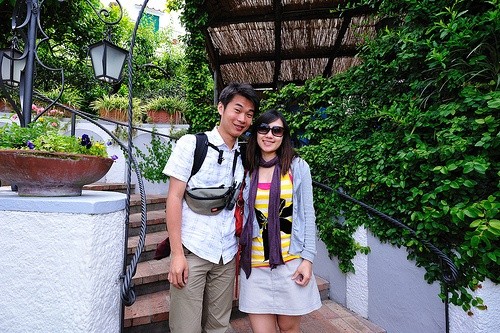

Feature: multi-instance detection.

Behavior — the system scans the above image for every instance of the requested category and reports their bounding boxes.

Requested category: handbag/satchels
[185,181,237,217]
[234,171,249,238]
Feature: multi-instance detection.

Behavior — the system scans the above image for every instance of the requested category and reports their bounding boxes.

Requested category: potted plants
[90,93,142,122]
[0,89,83,118]
[146,96,187,124]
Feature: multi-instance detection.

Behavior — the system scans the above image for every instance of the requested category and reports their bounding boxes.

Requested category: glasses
[258,123,285,137]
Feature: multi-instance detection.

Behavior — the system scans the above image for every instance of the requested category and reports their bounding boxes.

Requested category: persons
[166,80,262,333]
[238,110,316,333]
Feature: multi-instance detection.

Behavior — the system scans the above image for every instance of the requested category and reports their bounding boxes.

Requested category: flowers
[0,103,119,161]
